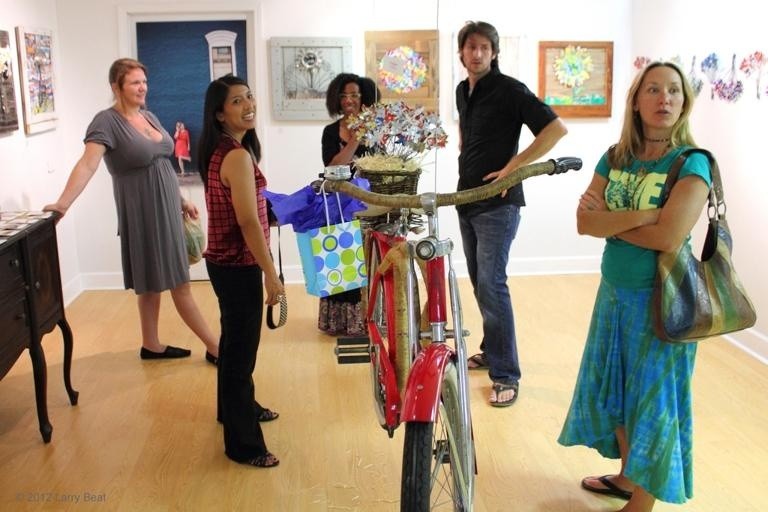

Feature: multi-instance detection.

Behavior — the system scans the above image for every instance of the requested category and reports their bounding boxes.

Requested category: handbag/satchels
[295,220,368,298]
[657,215,755,341]
[182,213,206,262]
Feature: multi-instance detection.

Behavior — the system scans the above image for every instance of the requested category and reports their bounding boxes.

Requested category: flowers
[345,101,448,161]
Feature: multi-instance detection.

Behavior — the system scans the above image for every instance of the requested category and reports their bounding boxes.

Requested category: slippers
[490,380,518,407]
[582,475,633,499]
[467,354,490,370]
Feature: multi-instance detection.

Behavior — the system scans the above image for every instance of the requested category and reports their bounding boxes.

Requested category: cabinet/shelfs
[0,209,79,443]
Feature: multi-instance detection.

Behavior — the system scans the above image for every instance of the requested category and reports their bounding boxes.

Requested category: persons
[558,61,713,512]
[318,72,386,336]
[197,73,286,467]
[42,57,219,365]
[174,121,192,175]
[456,20,567,407]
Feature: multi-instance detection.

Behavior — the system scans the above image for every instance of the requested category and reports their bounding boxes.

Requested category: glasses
[339,93,360,99]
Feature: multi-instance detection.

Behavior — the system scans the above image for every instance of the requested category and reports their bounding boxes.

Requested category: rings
[276,294,283,303]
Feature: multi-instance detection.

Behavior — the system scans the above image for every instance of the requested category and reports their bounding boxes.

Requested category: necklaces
[643,136,671,142]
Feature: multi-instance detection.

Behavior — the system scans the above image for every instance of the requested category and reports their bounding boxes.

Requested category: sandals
[259,409,279,421]
[241,452,280,467]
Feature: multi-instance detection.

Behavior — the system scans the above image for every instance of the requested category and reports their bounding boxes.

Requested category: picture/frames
[537,41,614,119]
[14,26,62,137]
[270,37,350,122]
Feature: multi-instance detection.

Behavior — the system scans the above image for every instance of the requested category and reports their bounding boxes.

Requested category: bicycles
[308,150,585,511]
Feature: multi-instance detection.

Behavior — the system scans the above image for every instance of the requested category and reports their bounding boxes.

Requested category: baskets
[358,169,422,222]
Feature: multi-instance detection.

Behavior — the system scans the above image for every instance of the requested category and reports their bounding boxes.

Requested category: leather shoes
[141,345,191,358]
[206,350,219,365]
